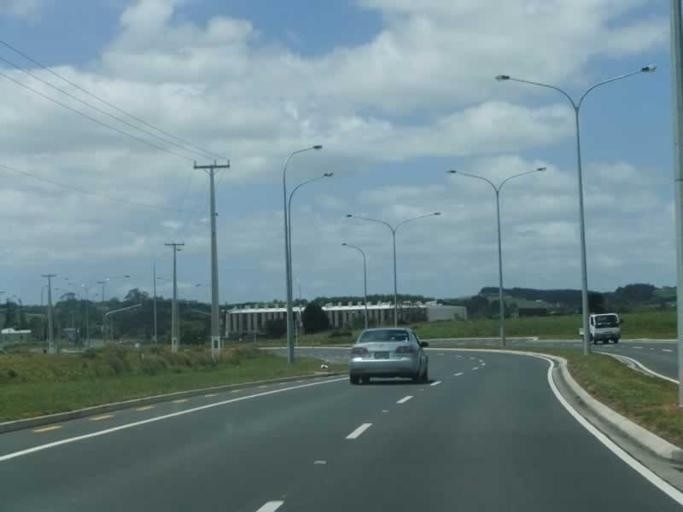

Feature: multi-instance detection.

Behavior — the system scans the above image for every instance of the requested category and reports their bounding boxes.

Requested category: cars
[348,327,429,386]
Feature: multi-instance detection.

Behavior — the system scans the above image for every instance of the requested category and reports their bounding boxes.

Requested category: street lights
[446,167,547,346]
[280,144,335,366]
[341,242,368,329]
[346,211,441,328]
[494,64,658,357]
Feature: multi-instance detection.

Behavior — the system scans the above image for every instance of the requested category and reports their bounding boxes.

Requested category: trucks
[579,312,624,345]
